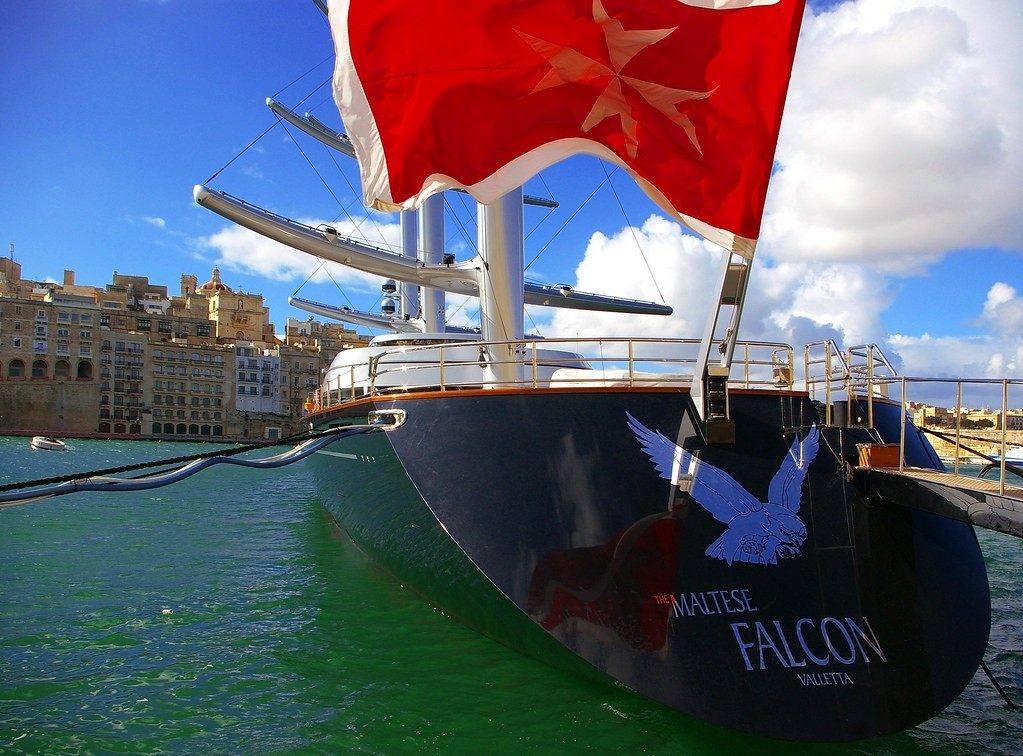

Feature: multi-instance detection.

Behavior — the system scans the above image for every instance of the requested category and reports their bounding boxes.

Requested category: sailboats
[193,0,991,744]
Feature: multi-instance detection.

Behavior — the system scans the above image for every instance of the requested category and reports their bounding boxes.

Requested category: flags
[324,0,805,257]
